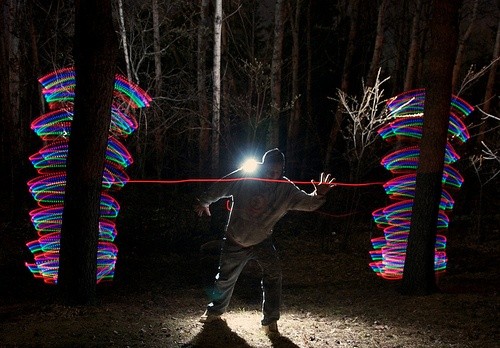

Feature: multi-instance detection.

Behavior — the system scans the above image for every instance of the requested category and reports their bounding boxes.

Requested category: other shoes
[263,320,280,339]
[200,310,220,322]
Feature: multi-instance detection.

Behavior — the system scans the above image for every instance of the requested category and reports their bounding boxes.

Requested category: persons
[193,149,337,338]
